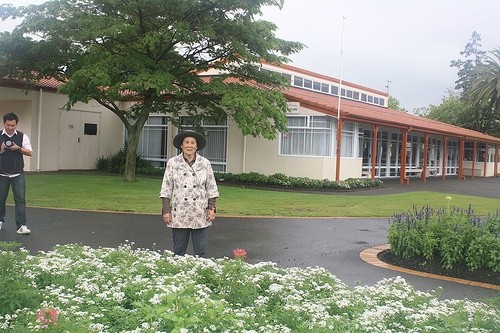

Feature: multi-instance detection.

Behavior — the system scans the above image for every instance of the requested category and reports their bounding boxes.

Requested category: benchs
[400,168,438,177]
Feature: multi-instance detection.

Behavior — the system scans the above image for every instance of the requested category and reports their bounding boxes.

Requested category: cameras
[4,139,12,147]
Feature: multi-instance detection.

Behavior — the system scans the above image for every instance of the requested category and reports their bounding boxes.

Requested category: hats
[172,130,206,151]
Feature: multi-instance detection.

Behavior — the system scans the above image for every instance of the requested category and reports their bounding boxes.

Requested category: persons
[158,128,220,258]
[0,112,33,234]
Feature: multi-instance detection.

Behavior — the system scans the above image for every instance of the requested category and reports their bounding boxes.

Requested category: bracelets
[18,147,21,153]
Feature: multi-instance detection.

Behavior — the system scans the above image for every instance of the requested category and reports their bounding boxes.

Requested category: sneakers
[0,221,3,231]
[16,225,31,235]
